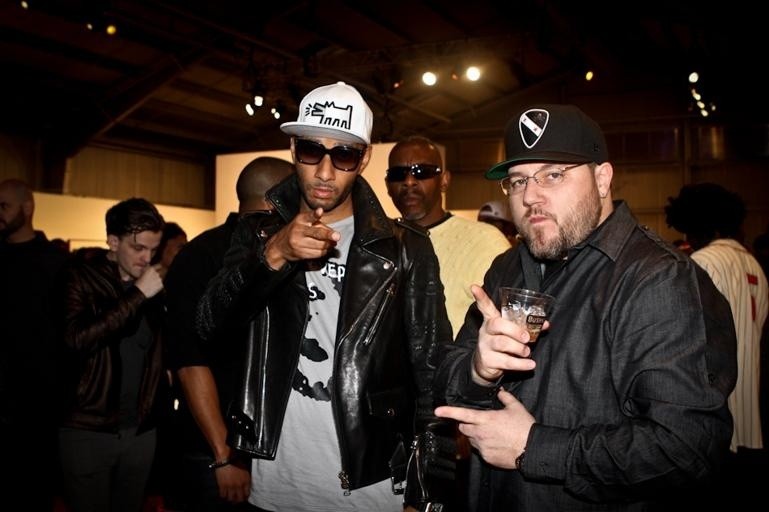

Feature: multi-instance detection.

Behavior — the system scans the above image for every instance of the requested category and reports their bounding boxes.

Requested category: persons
[387,135,514,339]
[0,158,294,512]
[198,80,452,512]
[435,105,768,511]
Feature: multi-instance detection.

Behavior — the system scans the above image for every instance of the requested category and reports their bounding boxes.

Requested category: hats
[484,105,609,179]
[480,201,511,221]
[280,81,373,144]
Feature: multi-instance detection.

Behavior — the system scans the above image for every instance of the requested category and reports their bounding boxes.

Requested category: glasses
[386,163,443,181]
[498,164,585,196]
[295,136,366,172]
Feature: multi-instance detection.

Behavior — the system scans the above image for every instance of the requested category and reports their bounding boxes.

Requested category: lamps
[244,102,256,117]
[271,103,286,120]
[253,80,265,107]
[451,66,464,81]
